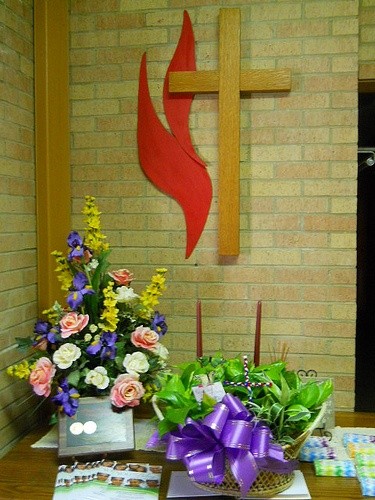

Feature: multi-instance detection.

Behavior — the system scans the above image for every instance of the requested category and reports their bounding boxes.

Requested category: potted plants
[151,343,334,498]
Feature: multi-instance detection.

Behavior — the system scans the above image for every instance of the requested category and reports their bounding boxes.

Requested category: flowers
[4,194,174,417]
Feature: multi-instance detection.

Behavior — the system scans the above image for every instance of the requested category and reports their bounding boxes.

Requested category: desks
[1,411,375,500]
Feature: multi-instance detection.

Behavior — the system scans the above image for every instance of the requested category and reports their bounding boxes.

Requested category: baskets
[148,389,329,497]
